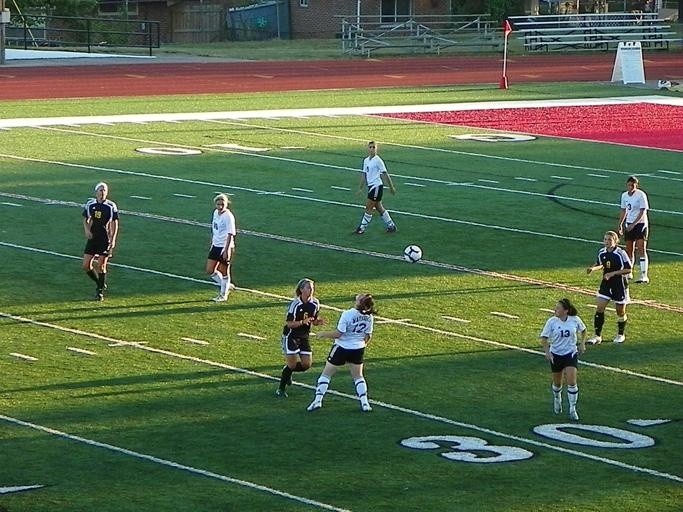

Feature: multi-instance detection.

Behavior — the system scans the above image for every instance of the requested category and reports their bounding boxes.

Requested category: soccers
[404,245,422,263]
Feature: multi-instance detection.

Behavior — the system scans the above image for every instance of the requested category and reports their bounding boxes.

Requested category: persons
[276,279,328,398]
[618,176,650,283]
[585,231,632,346]
[306,292,377,412]
[355,141,399,234]
[538,299,586,422]
[82,182,119,302]
[205,193,237,302]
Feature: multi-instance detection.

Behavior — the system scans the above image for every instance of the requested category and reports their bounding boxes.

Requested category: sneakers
[362,402,372,412]
[353,228,365,234]
[613,333,625,342]
[569,408,579,421]
[274,365,292,398]
[212,283,235,302]
[385,225,397,232]
[94,283,107,301]
[554,397,563,415]
[627,272,649,284]
[587,334,602,344]
[307,399,322,412]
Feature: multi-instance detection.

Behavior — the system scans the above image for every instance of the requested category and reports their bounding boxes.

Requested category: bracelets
[300,320,303,326]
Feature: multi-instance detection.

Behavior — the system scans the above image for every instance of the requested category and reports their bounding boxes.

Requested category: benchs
[333,13,509,58]
[509,10,683,52]
